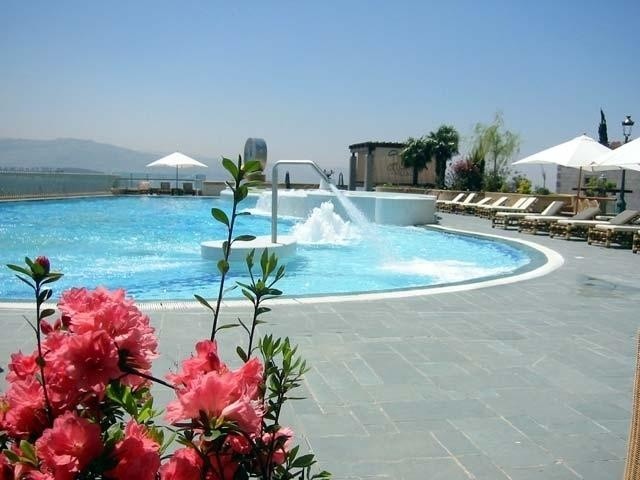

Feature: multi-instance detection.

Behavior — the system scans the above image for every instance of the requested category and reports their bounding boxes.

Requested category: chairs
[139,182,195,196]
[435,192,640,254]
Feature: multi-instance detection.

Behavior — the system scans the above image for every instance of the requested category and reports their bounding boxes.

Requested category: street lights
[617,116,635,214]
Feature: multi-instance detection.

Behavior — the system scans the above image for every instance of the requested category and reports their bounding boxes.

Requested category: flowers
[0,153,333,479]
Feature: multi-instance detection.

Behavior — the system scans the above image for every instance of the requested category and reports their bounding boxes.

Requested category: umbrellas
[146,150,208,189]
[511,133,627,215]
[593,137,640,172]
